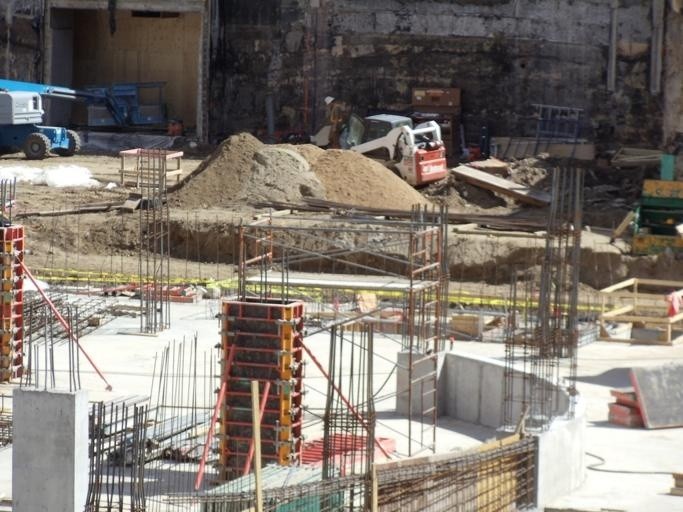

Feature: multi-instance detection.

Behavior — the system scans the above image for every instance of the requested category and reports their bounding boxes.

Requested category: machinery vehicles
[328,112,447,190]
[0,75,170,161]
